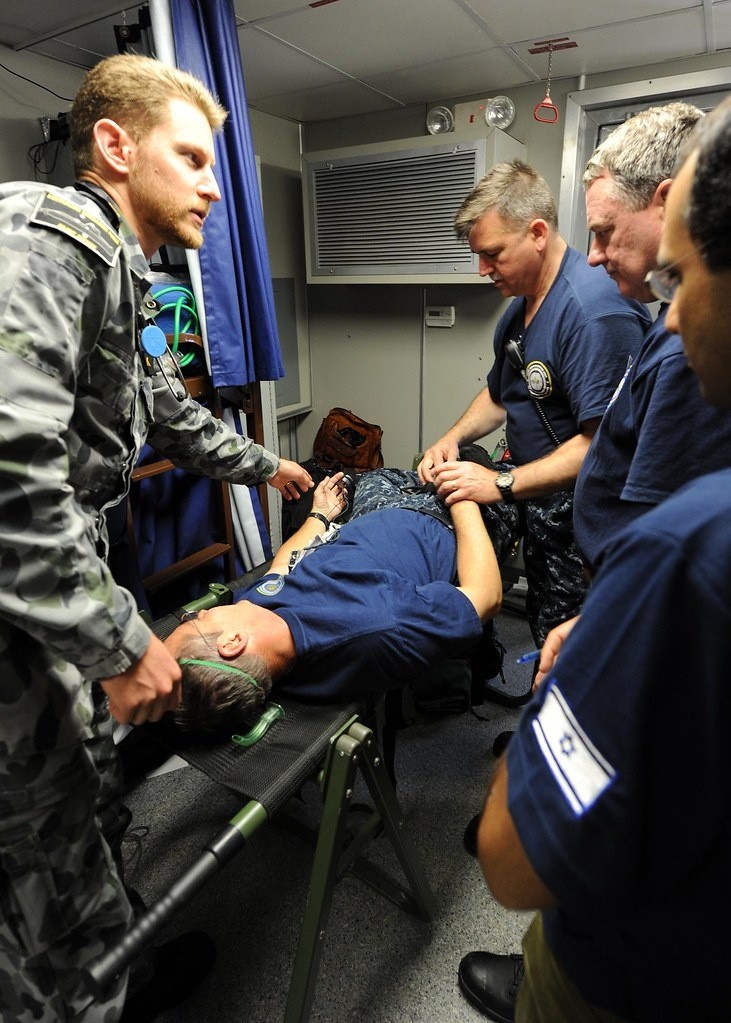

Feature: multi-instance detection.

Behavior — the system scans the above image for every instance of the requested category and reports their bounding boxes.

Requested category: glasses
[646,247,700,302]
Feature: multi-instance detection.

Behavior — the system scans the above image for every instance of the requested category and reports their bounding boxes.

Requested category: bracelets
[306,513,330,530]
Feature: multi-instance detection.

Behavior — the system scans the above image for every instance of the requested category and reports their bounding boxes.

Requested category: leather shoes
[459,950,527,1022]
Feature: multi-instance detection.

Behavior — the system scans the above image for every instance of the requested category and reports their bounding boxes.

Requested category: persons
[161,444,519,743]
[460,90,731,1023]
[415,159,653,756]
[0,55,315,1023]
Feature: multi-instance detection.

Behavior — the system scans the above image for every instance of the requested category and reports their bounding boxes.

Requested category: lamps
[427,96,515,135]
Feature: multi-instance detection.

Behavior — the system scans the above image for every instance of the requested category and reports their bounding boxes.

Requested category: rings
[286,482,292,486]
[337,496,342,502]
[452,481,457,490]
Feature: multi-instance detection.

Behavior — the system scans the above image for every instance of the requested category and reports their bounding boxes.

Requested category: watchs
[495,471,515,505]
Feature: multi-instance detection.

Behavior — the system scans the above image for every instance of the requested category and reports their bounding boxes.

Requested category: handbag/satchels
[312,407,385,474]
